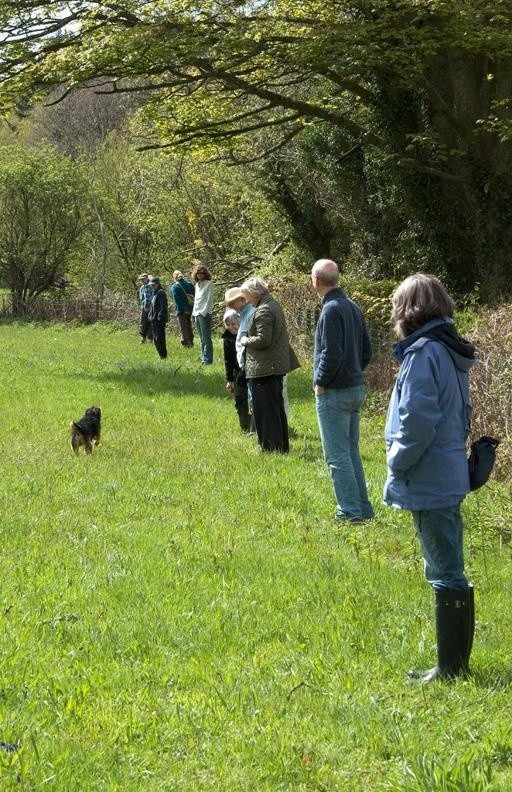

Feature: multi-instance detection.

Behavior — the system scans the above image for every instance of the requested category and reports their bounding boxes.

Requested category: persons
[239,276,301,454]
[223,286,292,437]
[311,258,376,527]
[189,264,215,367]
[148,277,168,360]
[381,269,478,687]
[222,309,253,433]
[139,273,155,345]
[171,270,195,347]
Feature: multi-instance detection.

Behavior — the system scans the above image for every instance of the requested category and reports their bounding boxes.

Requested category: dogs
[68,406,101,457]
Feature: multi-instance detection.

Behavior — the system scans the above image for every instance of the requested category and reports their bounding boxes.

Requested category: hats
[218,286,244,306]
[137,272,148,280]
[147,277,161,286]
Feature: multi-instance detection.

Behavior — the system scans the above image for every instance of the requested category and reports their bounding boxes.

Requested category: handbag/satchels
[467,435,499,491]
[186,292,195,307]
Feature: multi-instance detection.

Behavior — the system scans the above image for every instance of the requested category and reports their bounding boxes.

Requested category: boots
[405,579,476,685]
